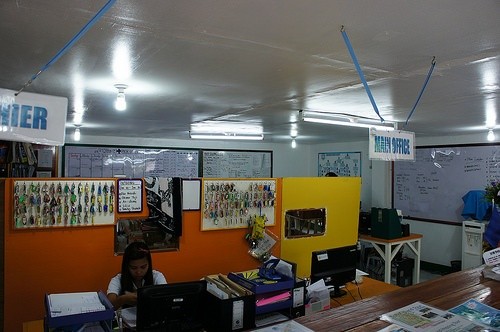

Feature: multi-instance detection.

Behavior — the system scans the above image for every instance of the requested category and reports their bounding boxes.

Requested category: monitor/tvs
[137,280,207,332]
[311,244,356,297]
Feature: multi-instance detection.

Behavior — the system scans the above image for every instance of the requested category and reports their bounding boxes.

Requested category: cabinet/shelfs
[44,272,306,332]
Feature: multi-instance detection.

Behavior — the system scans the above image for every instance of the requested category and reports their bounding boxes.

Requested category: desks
[330,276,400,309]
[360,232,423,285]
[273,265,500,332]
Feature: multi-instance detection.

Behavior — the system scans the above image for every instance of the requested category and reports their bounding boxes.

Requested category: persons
[107,241,167,309]
[325,172,338,177]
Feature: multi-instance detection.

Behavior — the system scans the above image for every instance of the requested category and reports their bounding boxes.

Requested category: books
[204,273,253,300]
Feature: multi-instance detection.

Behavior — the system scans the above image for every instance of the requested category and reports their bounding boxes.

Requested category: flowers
[481,179,500,204]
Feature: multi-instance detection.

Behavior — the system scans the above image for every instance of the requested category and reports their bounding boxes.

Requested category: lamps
[189,130,264,142]
[300,110,398,131]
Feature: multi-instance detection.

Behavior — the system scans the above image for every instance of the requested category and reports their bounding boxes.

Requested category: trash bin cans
[451,261,461,272]
[397,258,412,287]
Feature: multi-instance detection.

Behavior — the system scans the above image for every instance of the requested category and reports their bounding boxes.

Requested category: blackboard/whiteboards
[181,179,202,211]
[392,143,500,227]
[62,144,201,178]
[118,178,143,213]
[201,149,272,178]
